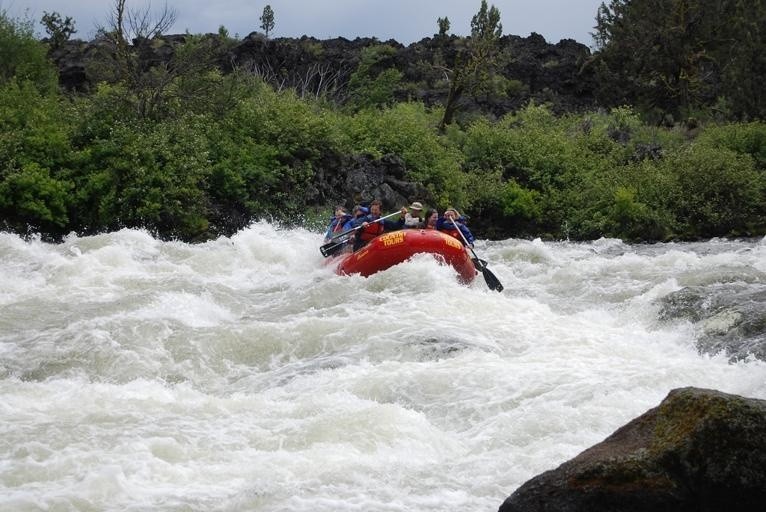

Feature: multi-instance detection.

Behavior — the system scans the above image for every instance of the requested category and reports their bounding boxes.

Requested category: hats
[408,201,424,212]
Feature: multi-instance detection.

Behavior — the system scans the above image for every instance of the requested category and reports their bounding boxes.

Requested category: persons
[323,200,474,254]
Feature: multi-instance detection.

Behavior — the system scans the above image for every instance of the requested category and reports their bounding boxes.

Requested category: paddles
[451,216,504,294]
[319,236,357,258]
[470,258,489,271]
[333,210,402,239]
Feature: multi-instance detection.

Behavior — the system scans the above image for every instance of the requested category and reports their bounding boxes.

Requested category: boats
[323,215,477,286]
